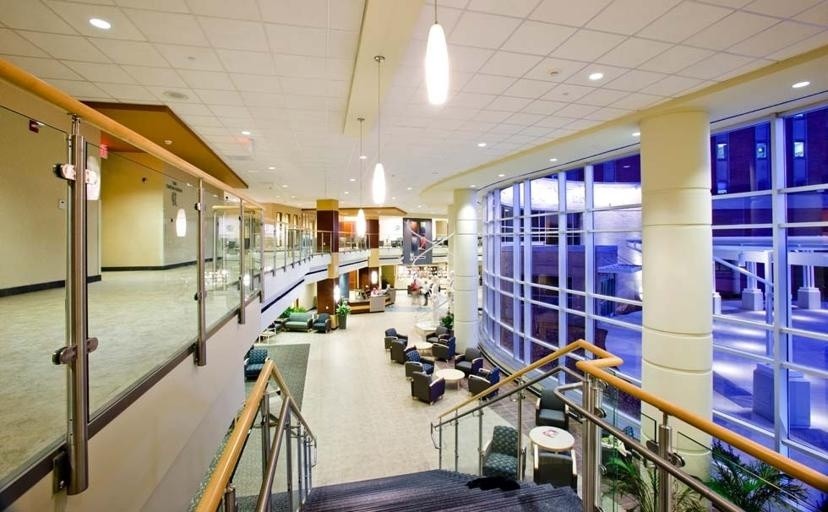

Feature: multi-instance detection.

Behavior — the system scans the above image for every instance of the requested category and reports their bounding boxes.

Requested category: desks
[529,426,576,454]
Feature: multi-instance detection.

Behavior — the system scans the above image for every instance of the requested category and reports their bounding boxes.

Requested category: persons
[410,276,436,306]
[371,286,378,295]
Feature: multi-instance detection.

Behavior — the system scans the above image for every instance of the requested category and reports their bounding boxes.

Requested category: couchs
[283,313,313,333]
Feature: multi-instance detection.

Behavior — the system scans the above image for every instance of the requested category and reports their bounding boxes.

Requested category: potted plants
[337,298,351,329]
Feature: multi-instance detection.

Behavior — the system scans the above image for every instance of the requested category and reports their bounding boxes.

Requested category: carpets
[245,343,310,429]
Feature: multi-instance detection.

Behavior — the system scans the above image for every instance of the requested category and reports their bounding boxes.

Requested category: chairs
[533,449,577,493]
[244,348,270,379]
[312,312,331,334]
[481,425,527,480]
[384,325,500,406]
[535,387,569,431]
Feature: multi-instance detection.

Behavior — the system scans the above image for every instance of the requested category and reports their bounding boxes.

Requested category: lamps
[356,56,387,239]
[426,0,451,106]
